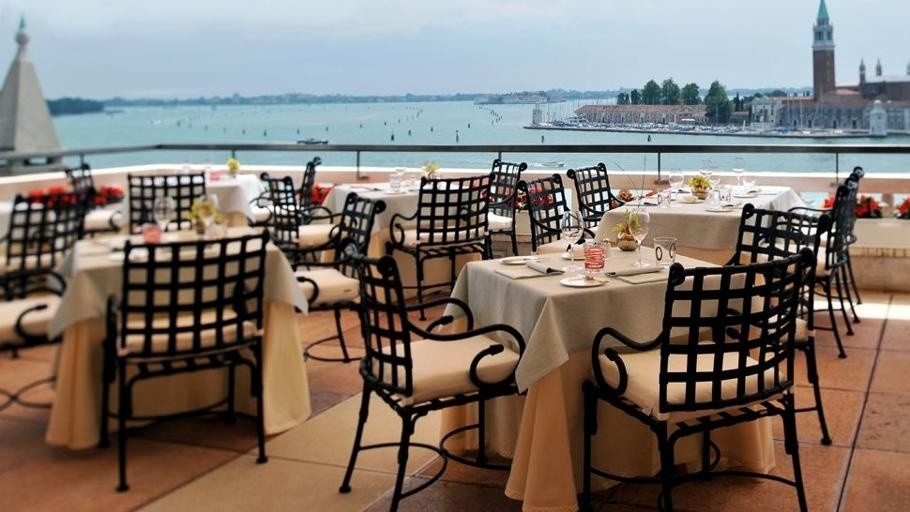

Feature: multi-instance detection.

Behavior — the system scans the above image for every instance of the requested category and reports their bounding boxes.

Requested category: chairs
[385,172,498,323]
[707,203,832,446]
[288,192,387,364]
[50,189,92,261]
[516,174,595,253]
[63,163,126,240]
[783,182,860,328]
[580,253,809,512]
[0,287,89,411]
[259,172,334,269]
[786,165,864,304]
[250,157,322,228]
[757,180,854,361]
[339,252,536,512]
[101,229,269,494]
[477,159,527,258]
[127,173,208,234]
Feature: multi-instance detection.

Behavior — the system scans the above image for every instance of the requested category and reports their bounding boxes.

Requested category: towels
[527,262,566,274]
[605,265,664,277]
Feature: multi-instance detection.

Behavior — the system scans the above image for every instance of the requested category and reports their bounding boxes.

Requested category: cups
[582,242,607,280]
[668,169,684,207]
[628,207,650,267]
[653,237,678,272]
[560,210,585,272]
[699,159,713,180]
[394,159,406,183]
[153,197,174,233]
[732,157,745,189]
[198,192,222,227]
[390,174,401,188]
[657,187,672,210]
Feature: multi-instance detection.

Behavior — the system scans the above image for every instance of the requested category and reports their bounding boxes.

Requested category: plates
[732,194,756,198]
[703,209,733,212]
[559,276,605,287]
[382,190,408,194]
[500,257,540,265]
[561,252,587,259]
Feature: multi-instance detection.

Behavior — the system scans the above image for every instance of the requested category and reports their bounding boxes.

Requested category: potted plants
[612,211,643,253]
[422,161,437,183]
[690,176,709,202]
[228,155,239,176]
[193,203,215,232]
